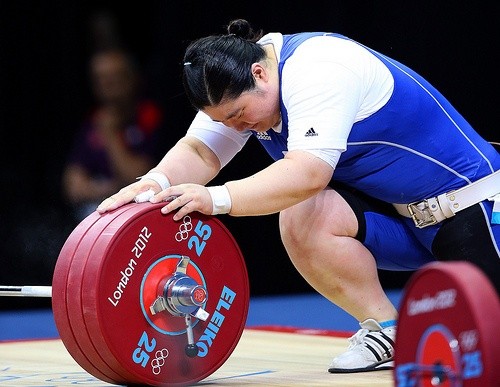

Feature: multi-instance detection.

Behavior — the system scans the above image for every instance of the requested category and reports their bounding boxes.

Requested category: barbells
[0,197,253,387]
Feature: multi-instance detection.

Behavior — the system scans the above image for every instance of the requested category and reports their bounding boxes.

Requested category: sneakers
[328,319,396,372]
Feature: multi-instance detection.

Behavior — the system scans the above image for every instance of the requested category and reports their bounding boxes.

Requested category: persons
[65,44,169,224]
[97,18,500,387]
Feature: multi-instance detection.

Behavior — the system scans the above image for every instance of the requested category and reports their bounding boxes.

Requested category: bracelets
[139,171,172,201]
[206,184,232,215]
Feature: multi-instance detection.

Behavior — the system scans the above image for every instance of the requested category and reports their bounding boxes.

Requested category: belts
[392,169,500,230]
